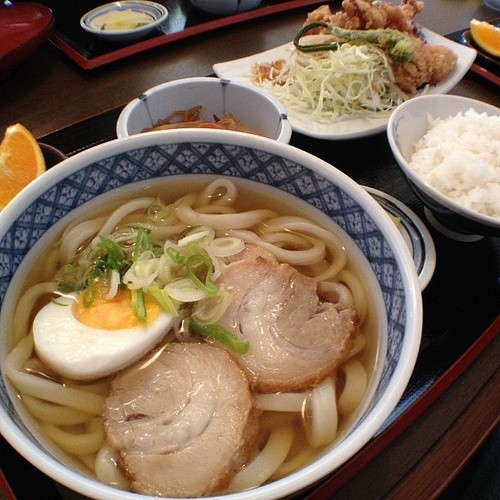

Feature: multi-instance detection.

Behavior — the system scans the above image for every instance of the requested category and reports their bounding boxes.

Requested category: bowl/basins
[386,93,500,245]
[0,127,425,500]
[79,0,169,40]
[114,76,293,146]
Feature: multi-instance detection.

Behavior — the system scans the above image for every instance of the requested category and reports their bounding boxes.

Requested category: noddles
[3,176,370,500]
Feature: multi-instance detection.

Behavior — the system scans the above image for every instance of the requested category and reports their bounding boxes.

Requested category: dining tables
[1,1,499,499]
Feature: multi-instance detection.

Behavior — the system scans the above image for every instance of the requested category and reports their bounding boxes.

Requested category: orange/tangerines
[0,123,45,213]
[469,15,500,57]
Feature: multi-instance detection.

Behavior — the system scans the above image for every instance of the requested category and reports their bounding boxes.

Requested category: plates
[0,1,56,62]
[462,28,500,71]
[357,183,437,294]
[37,142,68,171]
[211,18,479,140]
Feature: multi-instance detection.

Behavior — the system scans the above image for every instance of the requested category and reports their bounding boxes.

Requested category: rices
[406,107,500,220]
